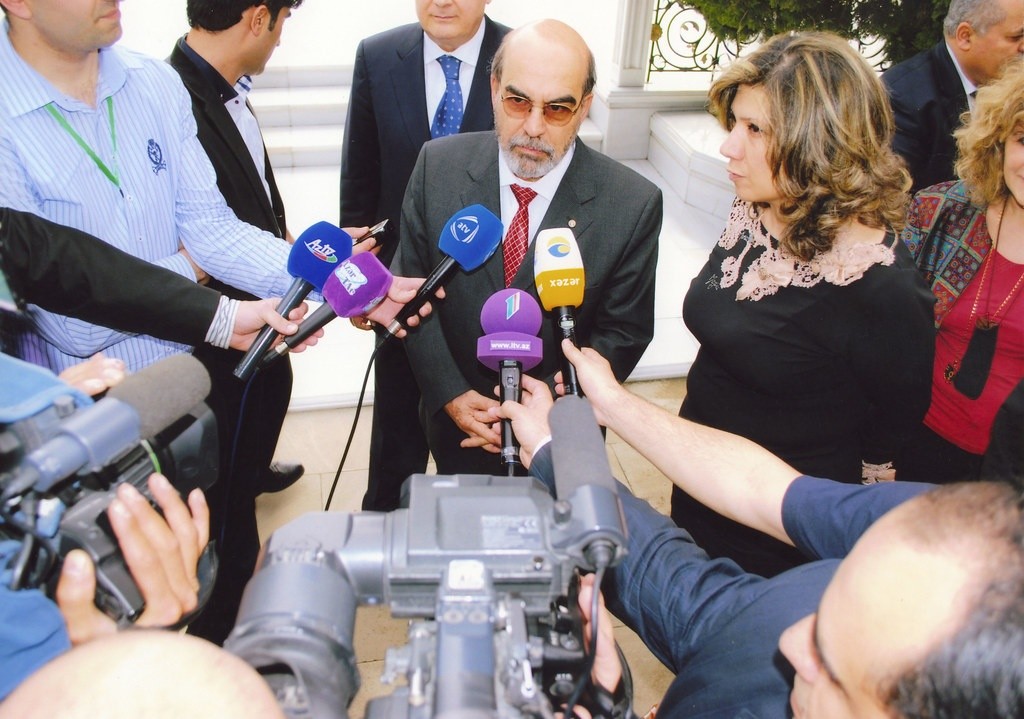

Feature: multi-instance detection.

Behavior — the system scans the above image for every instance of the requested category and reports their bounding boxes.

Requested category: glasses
[500,83,587,126]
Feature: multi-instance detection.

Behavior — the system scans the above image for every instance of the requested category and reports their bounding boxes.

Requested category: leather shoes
[268,463,304,493]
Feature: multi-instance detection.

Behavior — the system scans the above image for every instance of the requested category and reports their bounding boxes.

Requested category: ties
[970,91,978,98]
[431,55,463,139]
[503,184,539,288]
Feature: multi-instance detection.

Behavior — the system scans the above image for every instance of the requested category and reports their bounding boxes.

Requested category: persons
[489,370,1024,719]
[880,0,1024,195]
[900,58,1024,487]
[561,340,940,559]
[667,31,936,579]
[391,18,665,481]
[0,0,512,719]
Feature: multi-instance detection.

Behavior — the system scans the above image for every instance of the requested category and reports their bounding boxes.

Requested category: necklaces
[944,202,1024,385]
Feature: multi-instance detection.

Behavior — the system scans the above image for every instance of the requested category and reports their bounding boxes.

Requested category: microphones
[233,221,352,382]
[548,395,624,566]
[376,205,504,350]
[476,288,542,465]
[255,251,392,374]
[533,228,586,399]
[10,350,211,494]
